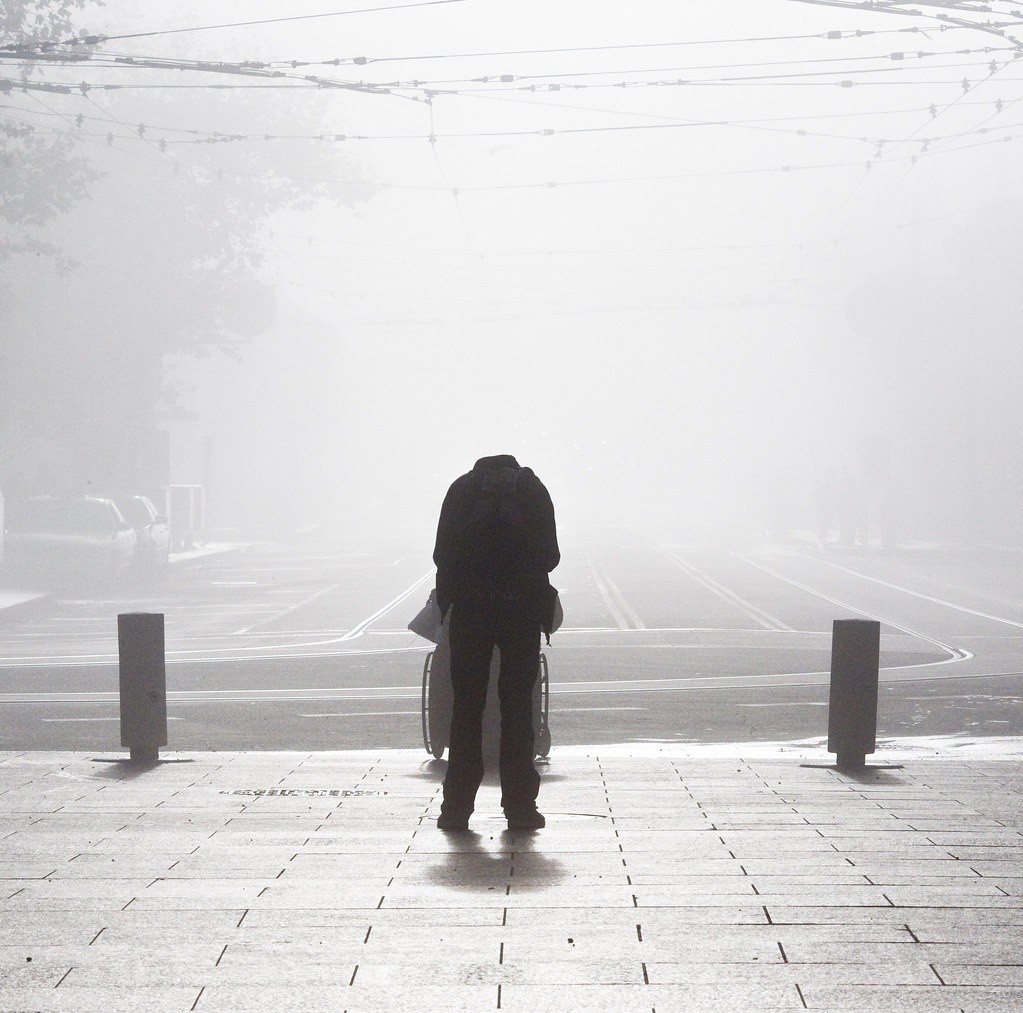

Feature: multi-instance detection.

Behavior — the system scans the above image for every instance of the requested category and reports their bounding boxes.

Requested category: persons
[434,454,561,833]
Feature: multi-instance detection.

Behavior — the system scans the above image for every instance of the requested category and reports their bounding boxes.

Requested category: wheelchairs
[421,651,552,765]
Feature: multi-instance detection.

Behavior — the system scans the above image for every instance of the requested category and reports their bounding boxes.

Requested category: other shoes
[436,808,471,830]
[507,810,545,830]
